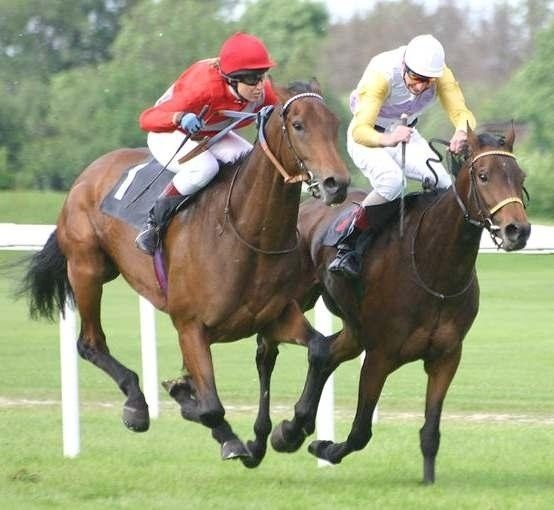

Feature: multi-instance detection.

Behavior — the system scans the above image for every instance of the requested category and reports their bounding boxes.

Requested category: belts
[374,117,417,133]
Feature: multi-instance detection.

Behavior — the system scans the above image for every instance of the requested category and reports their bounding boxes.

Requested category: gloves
[180,112,206,135]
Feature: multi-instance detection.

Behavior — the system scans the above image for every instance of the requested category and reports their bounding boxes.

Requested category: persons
[328,33,478,278]
[133,32,282,255]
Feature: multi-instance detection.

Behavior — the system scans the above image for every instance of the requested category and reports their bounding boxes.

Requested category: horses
[240,117,532,487]
[0,73,353,470]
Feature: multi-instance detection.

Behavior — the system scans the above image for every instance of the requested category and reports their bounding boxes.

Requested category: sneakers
[327,250,360,276]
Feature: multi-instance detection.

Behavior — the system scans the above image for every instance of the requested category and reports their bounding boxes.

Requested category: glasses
[239,72,266,86]
[407,70,436,85]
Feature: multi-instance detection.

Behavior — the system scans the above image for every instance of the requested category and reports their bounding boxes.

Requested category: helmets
[220,31,276,76]
[404,34,445,77]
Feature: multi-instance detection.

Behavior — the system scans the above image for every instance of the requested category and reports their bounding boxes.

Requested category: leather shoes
[135,221,161,256]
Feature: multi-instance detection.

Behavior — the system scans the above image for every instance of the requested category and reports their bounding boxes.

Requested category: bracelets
[176,111,186,124]
